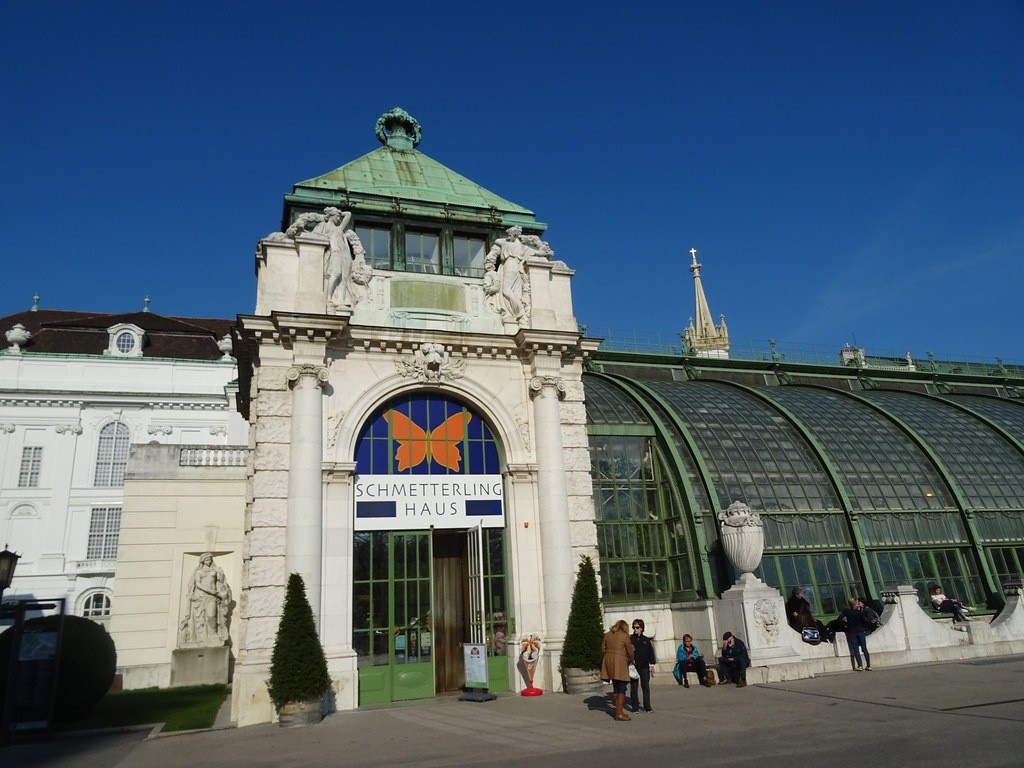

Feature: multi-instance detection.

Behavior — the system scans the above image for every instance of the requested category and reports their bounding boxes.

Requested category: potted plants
[262,572,333,730]
[558,554,606,693]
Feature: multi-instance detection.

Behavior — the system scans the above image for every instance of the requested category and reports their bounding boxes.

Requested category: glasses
[632,626,641,630]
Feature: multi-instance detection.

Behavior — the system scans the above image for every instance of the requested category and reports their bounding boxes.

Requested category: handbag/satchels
[802,627,820,644]
[705,670,716,685]
[628,662,639,680]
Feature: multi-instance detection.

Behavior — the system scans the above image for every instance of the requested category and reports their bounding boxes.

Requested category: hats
[723,632,731,640]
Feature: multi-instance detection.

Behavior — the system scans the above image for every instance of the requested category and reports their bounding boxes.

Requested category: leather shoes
[736,680,745,687]
[720,677,731,684]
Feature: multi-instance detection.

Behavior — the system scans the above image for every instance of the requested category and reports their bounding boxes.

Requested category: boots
[614,693,631,721]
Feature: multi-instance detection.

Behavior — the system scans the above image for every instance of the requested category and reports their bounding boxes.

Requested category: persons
[311,205,359,314]
[786,588,831,643]
[931,584,977,621]
[180,551,230,646]
[470,225,550,333]
[718,632,751,687]
[601,619,656,721]
[672,634,709,689]
[836,597,880,670]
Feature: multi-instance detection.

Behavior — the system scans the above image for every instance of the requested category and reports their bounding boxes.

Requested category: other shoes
[865,667,872,671]
[964,617,972,621]
[854,666,863,671]
[684,684,689,688]
[644,704,653,713]
[969,607,977,611]
[633,706,639,713]
[699,682,711,687]
